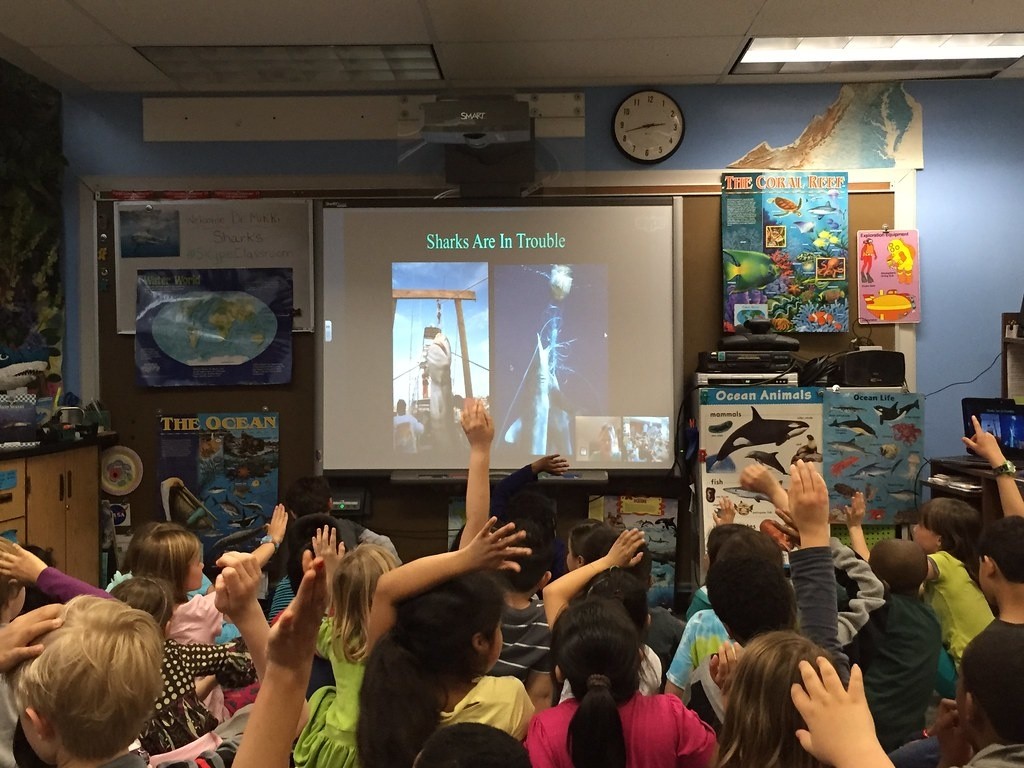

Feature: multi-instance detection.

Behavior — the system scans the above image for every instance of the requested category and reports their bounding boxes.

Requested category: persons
[1,400,1024,768]
[391,395,424,453]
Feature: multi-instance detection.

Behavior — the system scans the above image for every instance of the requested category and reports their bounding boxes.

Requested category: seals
[797,434,818,455]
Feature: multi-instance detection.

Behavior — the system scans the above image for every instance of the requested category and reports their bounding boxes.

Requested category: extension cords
[856,345,883,351]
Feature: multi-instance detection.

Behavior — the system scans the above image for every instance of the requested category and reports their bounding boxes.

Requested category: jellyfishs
[901,454,920,480]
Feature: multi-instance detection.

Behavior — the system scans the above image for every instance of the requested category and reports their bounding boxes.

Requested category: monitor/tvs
[978,405,1024,462]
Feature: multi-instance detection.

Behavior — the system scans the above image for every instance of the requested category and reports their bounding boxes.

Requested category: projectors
[422,100,532,148]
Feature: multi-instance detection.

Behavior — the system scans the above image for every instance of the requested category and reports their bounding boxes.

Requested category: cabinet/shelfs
[0,445,98,589]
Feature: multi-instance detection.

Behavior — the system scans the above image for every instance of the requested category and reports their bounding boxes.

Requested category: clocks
[610,89,686,164]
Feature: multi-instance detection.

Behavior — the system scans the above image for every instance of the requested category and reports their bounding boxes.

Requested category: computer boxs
[962,398,1016,456]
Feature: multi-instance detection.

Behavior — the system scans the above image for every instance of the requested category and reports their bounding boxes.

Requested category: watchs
[992,459,1017,479]
[259,534,280,554]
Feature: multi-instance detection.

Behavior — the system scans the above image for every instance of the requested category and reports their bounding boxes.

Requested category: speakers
[827,350,906,388]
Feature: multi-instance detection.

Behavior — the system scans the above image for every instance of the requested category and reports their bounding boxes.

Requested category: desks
[920,454,1024,525]
